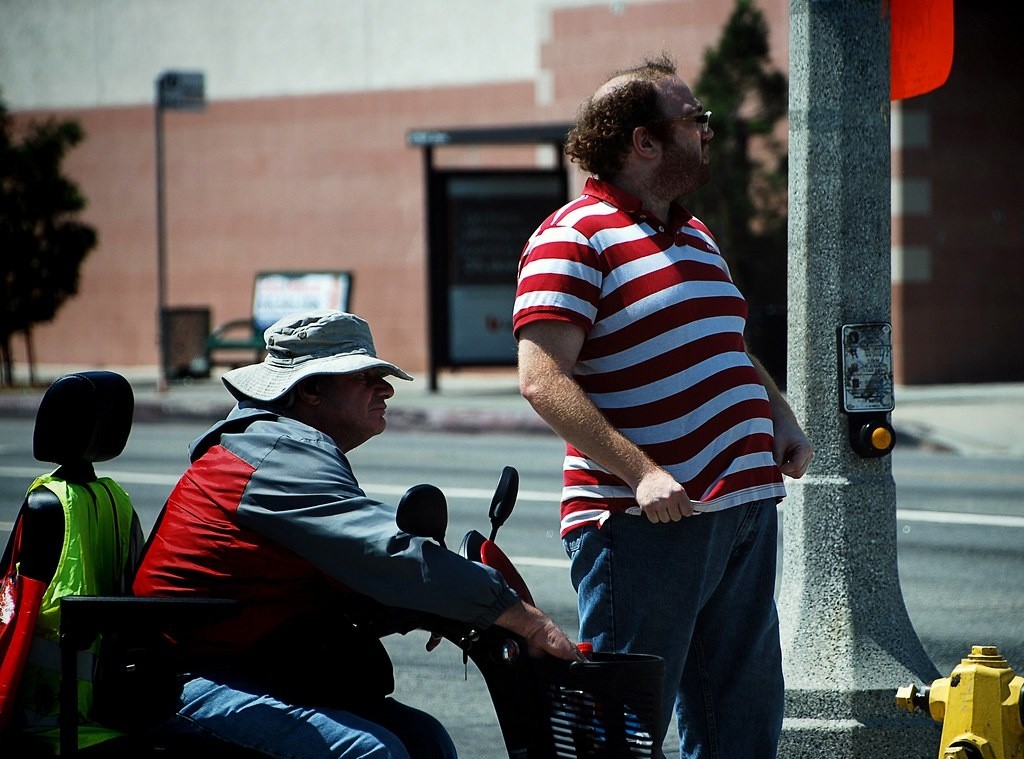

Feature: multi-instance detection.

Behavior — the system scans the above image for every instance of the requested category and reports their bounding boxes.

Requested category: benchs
[204,271,350,377]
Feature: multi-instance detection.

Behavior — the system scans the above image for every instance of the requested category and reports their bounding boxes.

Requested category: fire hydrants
[894,644,1024,759]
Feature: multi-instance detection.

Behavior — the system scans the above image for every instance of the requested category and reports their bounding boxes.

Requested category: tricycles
[0,367,680,759]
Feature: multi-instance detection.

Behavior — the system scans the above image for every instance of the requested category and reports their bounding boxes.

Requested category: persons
[93,310,589,758]
[510,51,816,759]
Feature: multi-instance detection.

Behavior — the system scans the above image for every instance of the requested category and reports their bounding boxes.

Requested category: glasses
[629,111,713,142]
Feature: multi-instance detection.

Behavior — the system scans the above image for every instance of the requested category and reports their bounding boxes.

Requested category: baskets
[519,652,669,758]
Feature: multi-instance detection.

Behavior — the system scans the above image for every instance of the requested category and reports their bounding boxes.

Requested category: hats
[220,308,415,403]
